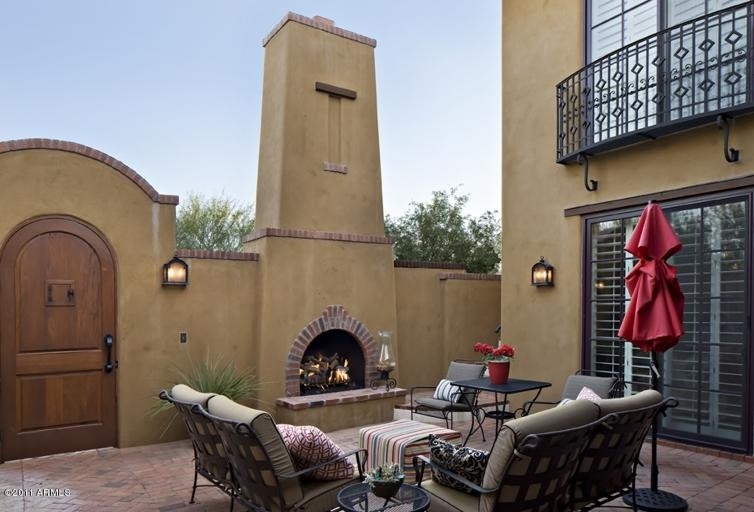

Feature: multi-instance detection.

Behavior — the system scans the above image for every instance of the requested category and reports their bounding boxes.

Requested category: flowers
[472,341,514,360]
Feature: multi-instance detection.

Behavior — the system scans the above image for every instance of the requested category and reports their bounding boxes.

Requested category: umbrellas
[618,198,690,512]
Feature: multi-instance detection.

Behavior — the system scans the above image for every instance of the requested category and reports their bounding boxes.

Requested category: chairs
[411,359,486,448]
[558,368,622,402]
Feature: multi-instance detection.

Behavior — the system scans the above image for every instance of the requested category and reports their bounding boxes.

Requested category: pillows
[429,434,491,497]
[433,378,464,402]
[274,423,354,478]
[577,386,602,405]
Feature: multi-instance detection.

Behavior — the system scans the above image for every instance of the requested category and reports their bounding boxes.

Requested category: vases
[488,361,509,385]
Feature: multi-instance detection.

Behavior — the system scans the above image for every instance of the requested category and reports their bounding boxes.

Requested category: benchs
[156,385,368,511]
[411,392,678,511]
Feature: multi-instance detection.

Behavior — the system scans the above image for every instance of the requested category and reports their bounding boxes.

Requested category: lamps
[162,254,188,286]
[532,256,554,287]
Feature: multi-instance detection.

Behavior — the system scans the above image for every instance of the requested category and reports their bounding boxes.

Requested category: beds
[451,376,551,448]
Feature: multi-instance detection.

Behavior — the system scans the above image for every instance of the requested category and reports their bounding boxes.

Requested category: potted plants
[364,461,403,499]
